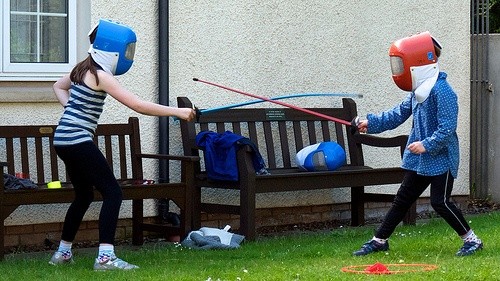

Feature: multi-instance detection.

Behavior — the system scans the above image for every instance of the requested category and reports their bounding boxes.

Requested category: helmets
[389,31,437,91]
[295,142,346,171]
[88,19,136,75]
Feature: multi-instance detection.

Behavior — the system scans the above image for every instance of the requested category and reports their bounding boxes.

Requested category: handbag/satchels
[195,130,271,181]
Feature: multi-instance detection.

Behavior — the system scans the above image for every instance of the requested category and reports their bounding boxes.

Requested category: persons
[48,19,197,270]
[351,31,483,256]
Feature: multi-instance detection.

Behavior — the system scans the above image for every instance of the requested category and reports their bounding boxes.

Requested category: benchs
[177,97,417,242]
[0,117,200,266]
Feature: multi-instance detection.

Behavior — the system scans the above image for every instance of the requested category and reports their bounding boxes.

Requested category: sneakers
[94,254,140,271]
[354,239,389,256]
[49,252,76,267]
[455,241,483,256]
